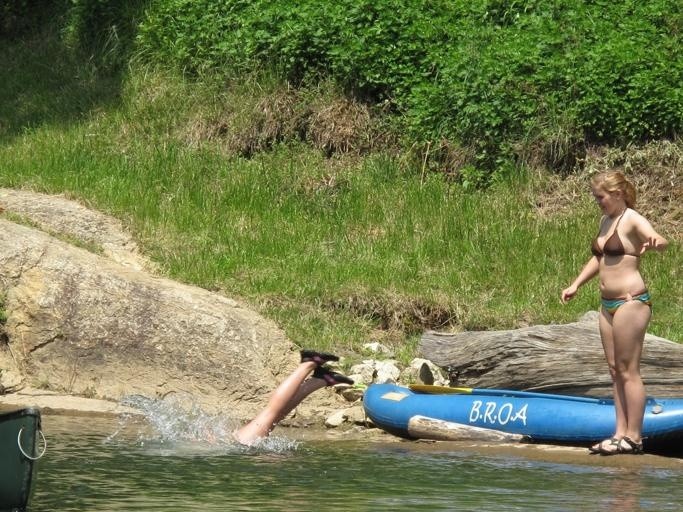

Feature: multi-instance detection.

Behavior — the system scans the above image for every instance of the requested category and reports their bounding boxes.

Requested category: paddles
[410,383,602,403]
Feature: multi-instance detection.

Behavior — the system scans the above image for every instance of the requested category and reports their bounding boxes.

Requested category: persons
[561,170,671,458]
[228,349,356,446]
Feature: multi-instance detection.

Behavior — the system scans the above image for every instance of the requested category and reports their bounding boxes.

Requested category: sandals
[590,436,622,456]
[599,435,645,456]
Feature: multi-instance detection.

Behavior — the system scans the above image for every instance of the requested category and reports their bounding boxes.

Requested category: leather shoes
[301,350,340,366]
[311,365,354,387]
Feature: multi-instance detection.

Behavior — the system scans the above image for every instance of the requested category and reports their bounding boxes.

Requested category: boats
[0,406,48,512]
[358,378,682,449]
